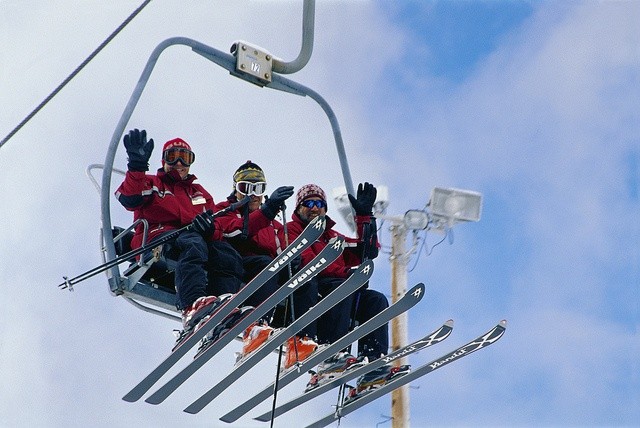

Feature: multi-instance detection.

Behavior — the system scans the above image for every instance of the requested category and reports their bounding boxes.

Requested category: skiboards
[120,213,345,409]
[254,313,507,428]
[181,262,426,423]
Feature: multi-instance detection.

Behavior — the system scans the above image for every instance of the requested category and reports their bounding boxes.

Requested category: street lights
[336,180,485,428]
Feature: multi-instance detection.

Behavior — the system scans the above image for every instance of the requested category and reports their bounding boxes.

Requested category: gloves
[193,208,216,241]
[122,128,155,171]
[260,185,295,220]
[282,253,302,275]
[346,269,369,292]
[348,181,377,215]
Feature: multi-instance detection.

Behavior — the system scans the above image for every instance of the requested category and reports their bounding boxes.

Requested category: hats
[161,137,191,167]
[296,184,328,213]
[234,160,264,191]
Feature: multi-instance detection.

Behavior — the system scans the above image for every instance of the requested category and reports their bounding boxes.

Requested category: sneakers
[205,305,255,344]
[356,352,400,390]
[182,295,217,331]
[284,335,318,369]
[308,342,357,387]
[243,319,275,357]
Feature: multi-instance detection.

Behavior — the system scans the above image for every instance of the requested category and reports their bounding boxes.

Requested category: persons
[115,129,242,341]
[278,182,409,388]
[216,160,319,369]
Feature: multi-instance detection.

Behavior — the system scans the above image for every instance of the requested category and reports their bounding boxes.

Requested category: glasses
[300,199,326,208]
[235,180,267,196]
[162,147,195,166]
